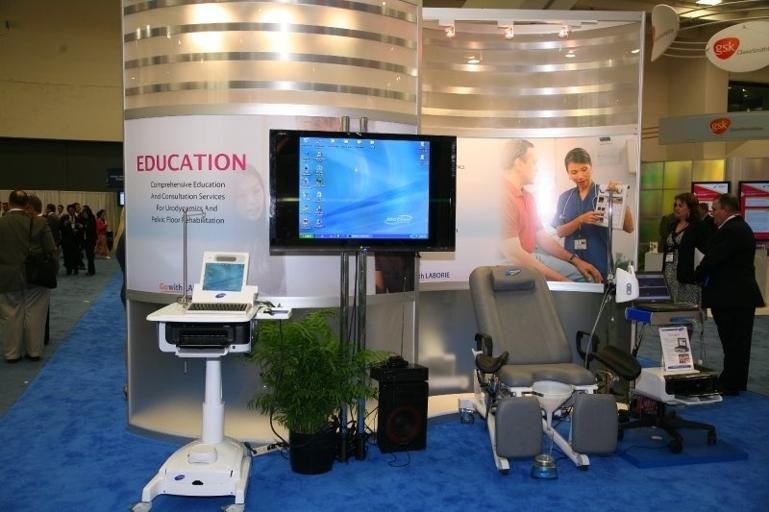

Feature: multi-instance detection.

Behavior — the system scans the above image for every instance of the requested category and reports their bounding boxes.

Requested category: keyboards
[184,302,250,314]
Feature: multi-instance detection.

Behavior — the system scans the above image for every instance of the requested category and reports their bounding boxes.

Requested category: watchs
[568,253,579,263]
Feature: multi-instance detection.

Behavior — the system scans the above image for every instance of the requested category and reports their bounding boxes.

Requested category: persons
[698,194,757,397]
[1,186,111,365]
[492,139,601,281]
[656,191,717,367]
[375,249,405,293]
[225,165,288,296]
[549,148,635,282]
[113,206,128,398]
[697,202,715,319]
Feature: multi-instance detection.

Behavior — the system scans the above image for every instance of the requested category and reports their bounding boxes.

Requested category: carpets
[0,268,768,511]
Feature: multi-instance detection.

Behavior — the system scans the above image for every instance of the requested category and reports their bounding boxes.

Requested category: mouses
[263,308,272,313]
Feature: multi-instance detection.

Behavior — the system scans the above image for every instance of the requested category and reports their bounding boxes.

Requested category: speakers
[378,381,429,454]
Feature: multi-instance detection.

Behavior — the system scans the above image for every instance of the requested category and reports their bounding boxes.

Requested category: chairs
[462,265,620,478]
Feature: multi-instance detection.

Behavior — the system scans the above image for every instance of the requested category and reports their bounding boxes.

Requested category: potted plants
[235,308,395,476]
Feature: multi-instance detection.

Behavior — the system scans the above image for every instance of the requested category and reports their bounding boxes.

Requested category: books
[593,183,630,230]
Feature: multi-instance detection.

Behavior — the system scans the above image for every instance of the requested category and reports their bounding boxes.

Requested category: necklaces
[679,222,688,230]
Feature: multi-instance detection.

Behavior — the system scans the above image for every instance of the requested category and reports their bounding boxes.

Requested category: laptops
[633,271,699,312]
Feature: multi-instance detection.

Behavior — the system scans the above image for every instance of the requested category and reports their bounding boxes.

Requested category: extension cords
[273,441,288,453]
[250,444,279,457]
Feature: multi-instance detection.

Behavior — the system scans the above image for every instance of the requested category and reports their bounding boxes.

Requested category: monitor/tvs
[117,189,125,207]
[198,251,249,295]
[268,129,458,256]
